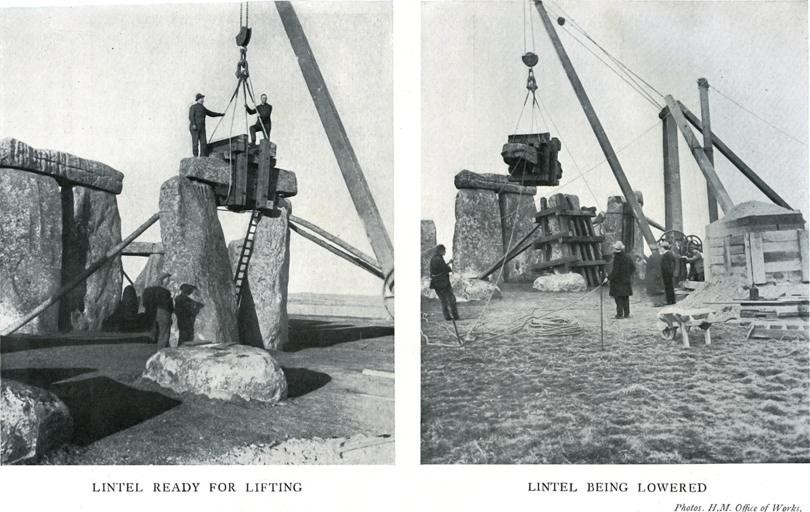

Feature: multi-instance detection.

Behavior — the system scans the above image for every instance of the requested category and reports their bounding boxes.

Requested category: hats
[160,272,171,281]
[612,240,626,251]
[661,241,670,247]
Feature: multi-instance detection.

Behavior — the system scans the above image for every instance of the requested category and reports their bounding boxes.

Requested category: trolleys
[656,303,739,350]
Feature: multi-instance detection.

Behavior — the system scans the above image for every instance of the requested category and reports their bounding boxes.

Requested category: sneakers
[611,314,623,319]
[623,314,633,318]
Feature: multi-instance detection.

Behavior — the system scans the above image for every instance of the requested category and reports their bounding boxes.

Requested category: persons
[429,244,458,321]
[645,241,704,305]
[155,272,174,352]
[188,92,227,157]
[603,240,635,319]
[243,93,272,147]
